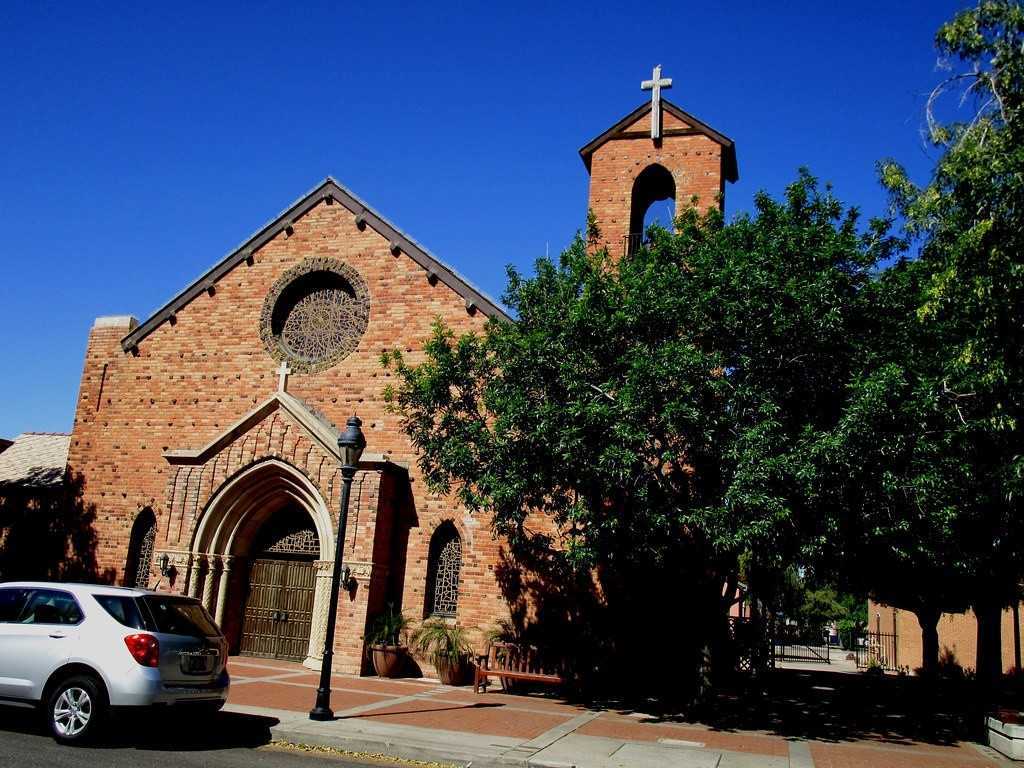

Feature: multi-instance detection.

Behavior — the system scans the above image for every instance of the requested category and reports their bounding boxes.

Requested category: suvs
[1,582,231,749]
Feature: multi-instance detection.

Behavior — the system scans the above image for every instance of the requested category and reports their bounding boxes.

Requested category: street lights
[308,412,370,723]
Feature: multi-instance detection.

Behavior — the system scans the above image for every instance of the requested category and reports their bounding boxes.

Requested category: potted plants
[363,602,417,678]
[483,619,538,696]
[411,615,484,685]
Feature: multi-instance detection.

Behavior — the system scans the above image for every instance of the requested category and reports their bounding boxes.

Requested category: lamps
[160,553,172,577]
[340,564,353,591]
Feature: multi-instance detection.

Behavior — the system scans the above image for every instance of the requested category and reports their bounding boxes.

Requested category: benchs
[473,642,581,697]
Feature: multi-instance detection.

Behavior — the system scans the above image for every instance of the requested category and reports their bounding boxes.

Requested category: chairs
[33,605,61,623]
